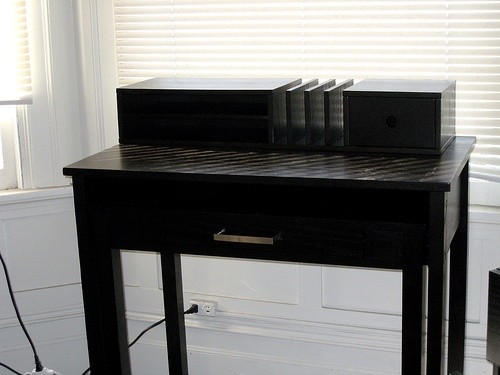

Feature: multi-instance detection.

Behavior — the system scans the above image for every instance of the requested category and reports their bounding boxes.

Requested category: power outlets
[188,300,218,317]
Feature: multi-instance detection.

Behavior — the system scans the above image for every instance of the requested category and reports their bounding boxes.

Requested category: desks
[63,134,479,375]
